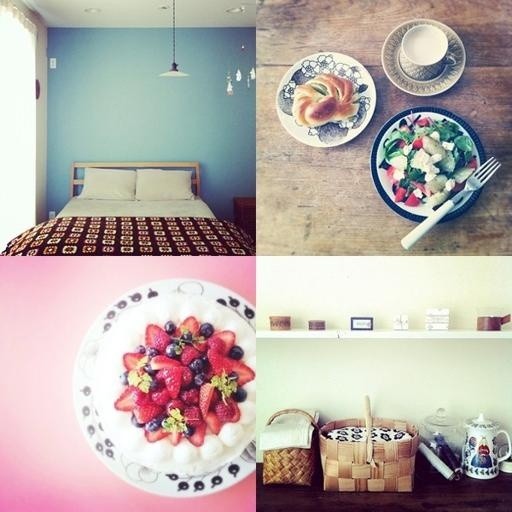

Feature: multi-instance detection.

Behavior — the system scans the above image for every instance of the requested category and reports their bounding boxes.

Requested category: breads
[290,73,360,128]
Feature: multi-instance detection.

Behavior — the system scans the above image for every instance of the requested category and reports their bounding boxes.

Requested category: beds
[2,161,256,255]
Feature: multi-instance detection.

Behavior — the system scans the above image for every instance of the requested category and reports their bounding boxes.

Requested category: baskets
[262,407,318,487]
[319,396,421,494]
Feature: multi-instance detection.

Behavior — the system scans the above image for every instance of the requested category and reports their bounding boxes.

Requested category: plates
[71,278,255,498]
[380,16,466,96]
[275,52,378,148]
[370,104,487,224]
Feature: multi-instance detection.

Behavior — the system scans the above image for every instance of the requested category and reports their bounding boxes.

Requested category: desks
[256,460,512,512]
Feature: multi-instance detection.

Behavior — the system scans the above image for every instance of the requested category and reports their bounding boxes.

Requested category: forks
[400,156,502,250]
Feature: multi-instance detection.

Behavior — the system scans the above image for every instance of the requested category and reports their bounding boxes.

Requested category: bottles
[418,408,463,486]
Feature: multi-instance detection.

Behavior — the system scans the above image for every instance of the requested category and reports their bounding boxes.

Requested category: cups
[462,412,512,480]
[400,24,458,79]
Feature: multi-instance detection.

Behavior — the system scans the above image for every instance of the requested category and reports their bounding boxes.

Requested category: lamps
[158,1,189,79]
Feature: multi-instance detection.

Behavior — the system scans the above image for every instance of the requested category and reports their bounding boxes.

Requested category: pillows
[77,167,198,202]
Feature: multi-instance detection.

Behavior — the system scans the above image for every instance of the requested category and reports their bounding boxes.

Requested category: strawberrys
[113,316,256,446]
